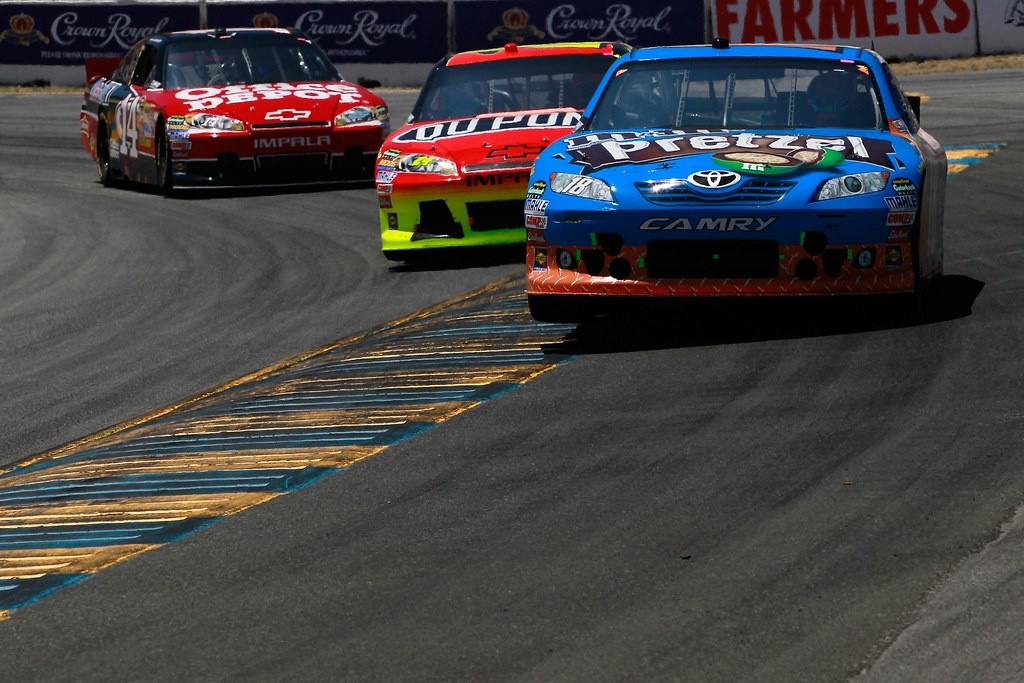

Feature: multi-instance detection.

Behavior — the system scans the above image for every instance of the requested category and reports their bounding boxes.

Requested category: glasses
[812,95,835,106]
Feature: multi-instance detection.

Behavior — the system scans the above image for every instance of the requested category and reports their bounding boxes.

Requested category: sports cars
[374,40,659,262]
[524,37,949,323]
[80,28,391,198]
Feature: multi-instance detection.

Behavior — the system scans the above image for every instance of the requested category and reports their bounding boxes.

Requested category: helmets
[807,75,844,112]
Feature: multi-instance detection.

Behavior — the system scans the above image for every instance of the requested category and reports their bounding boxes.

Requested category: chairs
[492,90,518,113]
[152,63,186,88]
